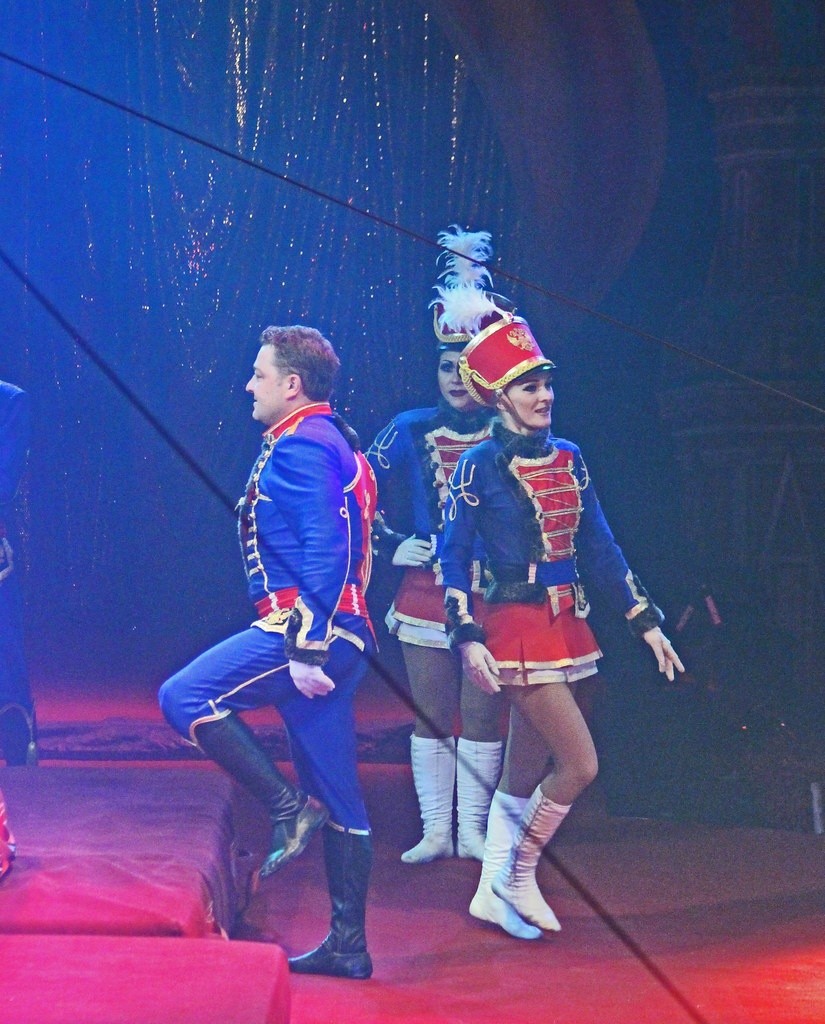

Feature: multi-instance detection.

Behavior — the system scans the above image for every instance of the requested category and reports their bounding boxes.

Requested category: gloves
[391,533,432,567]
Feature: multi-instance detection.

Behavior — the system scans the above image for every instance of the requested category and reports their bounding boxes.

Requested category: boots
[456,737,503,861]
[287,819,372,978]
[400,734,456,864]
[469,785,573,939]
[181,708,330,881]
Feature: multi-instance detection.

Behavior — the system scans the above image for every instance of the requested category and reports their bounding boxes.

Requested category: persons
[160,325,382,980]
[0,382,37,885]
[363,224,686,943]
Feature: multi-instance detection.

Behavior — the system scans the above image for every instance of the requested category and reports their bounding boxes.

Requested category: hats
[433,286,558,406]
[428,223,514,343]
